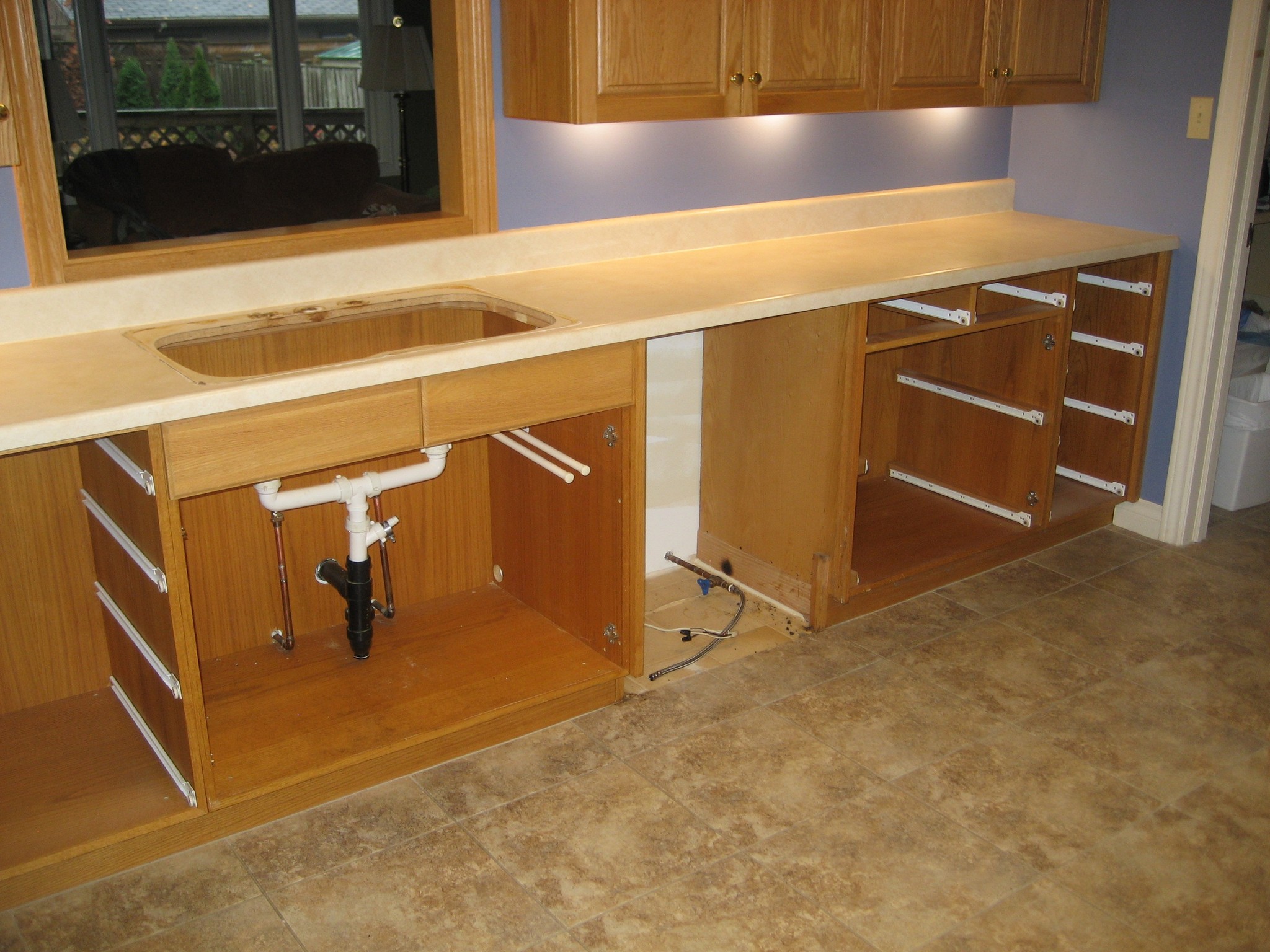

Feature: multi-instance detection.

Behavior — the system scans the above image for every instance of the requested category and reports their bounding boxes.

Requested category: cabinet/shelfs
[699,253,1171,632]
[880,1,1109,111]
[501,1,882,124]
[1,338,649,913]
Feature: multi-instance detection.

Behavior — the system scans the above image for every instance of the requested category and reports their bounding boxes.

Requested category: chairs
[61,137,380,241]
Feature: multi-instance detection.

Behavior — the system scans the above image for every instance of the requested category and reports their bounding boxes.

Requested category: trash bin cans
[1211,373,1270,512]
